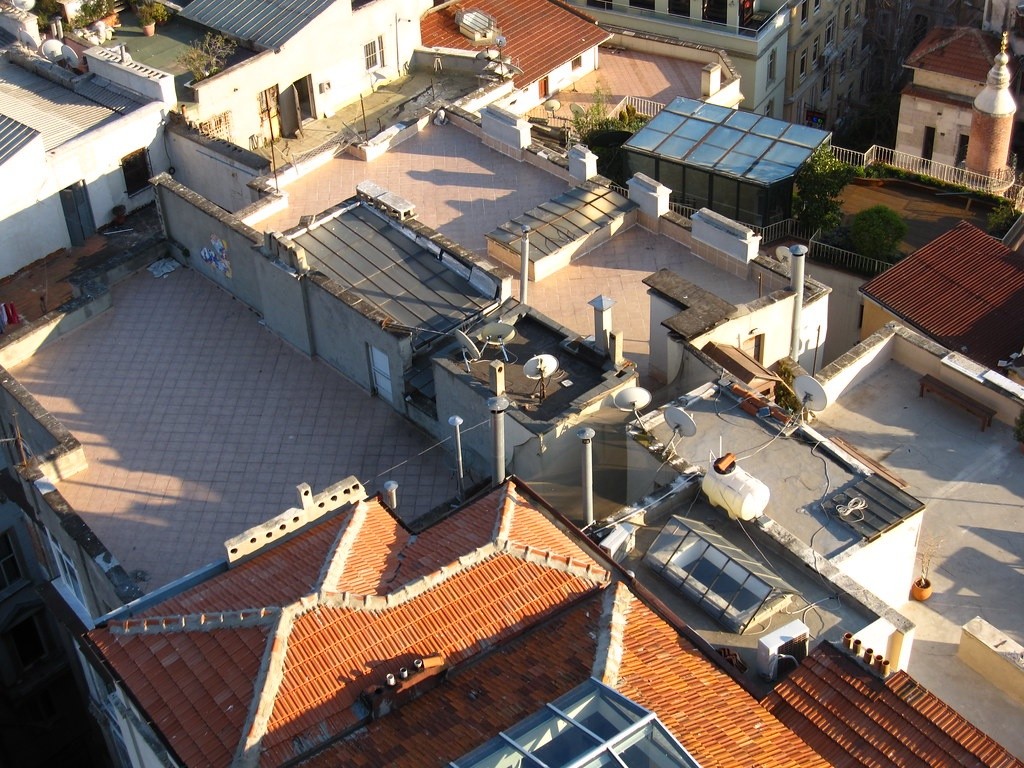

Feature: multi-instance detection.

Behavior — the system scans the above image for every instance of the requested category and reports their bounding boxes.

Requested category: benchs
[919,374,997,432]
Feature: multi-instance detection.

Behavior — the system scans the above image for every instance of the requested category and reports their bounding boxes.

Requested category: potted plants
[112,205,127,225]
[912,536,945,600]
[176,32,237,103]
[74,0,116,31]
[136,0,168,36]
[1013,404,1024,457]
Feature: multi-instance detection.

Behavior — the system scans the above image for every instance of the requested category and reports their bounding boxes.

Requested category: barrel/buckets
[701,453,771,521]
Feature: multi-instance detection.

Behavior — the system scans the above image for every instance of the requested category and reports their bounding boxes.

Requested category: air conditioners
[757,619,809,682]
[835,118,842,131]
